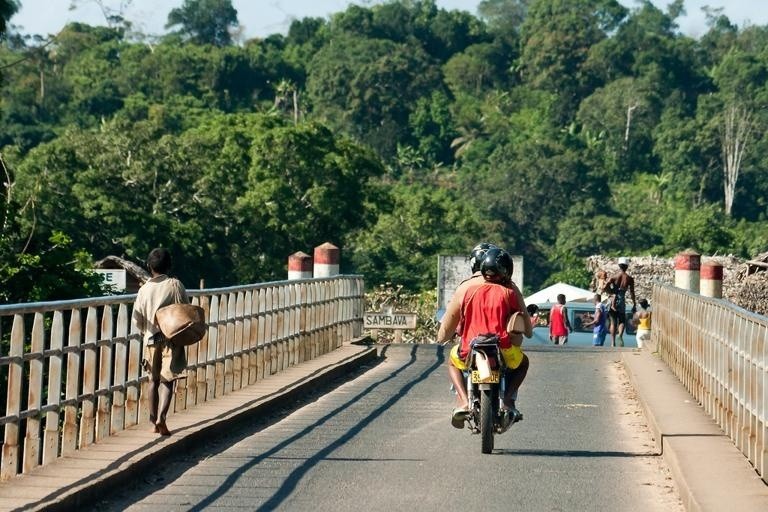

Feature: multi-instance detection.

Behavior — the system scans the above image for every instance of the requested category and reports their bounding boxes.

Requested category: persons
[582,293,607,345]
[593,271,618,312]
[547,293,572,346]
[131,246,191,436]
[435,241,534,428]
[446,247,536,421]
[602,262,637,347]
[628,298,652,348]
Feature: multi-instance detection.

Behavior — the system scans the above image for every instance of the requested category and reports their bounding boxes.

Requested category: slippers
[451,409,470,429]
[504,408,523,421]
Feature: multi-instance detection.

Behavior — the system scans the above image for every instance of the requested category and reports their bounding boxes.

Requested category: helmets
[480,248,514,282]
[469,243,496,273]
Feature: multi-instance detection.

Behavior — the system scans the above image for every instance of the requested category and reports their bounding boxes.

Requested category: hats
[618,257,630,265]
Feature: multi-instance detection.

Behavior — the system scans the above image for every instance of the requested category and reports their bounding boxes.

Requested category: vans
[514,301,656,349]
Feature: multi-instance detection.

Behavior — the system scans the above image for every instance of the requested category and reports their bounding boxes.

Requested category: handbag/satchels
[156,302,206,348]
[507,310,526,339]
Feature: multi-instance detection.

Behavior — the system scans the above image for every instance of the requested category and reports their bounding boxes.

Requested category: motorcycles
[435,304,539,457]
